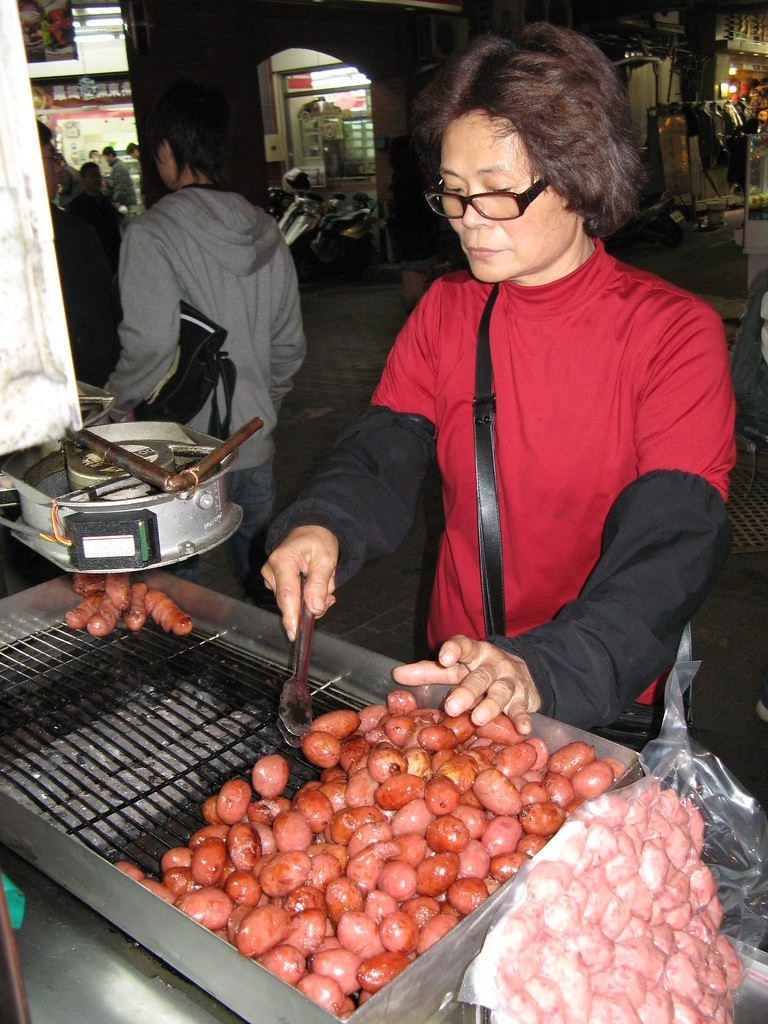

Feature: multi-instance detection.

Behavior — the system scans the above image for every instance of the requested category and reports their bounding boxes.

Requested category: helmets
[280,167,312,194]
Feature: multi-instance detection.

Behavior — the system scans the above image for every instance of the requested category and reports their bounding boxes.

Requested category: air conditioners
[414,13,469,61]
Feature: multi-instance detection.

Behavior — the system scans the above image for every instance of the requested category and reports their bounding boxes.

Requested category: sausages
[111,689,627,1021]
[64,572,193,638]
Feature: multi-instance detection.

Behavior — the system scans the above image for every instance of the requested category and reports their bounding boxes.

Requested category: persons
[102,80,308,616]
[262,24,739,754]
[55,153,86,210]
[126,143,145,204]
[37,120,122,389]
[102,146,137,217]
[66,163,122,275]
[726,118,759,184]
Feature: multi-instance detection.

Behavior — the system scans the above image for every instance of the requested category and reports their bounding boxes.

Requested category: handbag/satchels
[132,299,236,439]
[586,701,693,754]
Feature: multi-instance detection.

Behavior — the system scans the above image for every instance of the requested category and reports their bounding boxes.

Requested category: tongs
[278,572,314,748]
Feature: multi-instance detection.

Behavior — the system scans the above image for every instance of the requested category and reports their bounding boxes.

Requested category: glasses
[421,172,552,221]
[42,151,64,166]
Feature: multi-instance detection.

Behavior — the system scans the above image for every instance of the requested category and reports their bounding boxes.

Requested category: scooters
[601,189,688,248]
[265,169,379,284]
